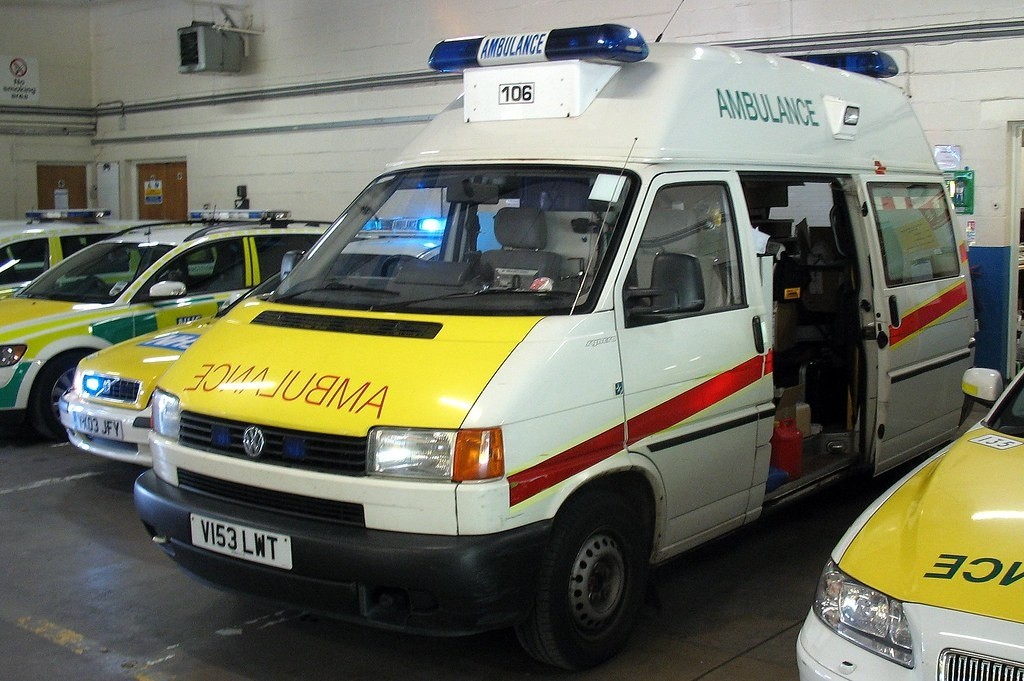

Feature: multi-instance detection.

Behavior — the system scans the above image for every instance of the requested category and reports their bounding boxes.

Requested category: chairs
[480,208,559,284]
[207,245,244,293]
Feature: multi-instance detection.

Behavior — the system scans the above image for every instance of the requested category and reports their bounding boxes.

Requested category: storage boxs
[775,384,814,439]
[775,302,798,354]
[796,218,844,313]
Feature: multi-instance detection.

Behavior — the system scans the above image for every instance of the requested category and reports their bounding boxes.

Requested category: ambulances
[130,25,979,674]
[56,215,447,470]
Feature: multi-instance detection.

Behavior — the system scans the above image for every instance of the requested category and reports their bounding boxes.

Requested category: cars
[793,355,1023,680]
[0,207,219,290]
[1,182,334,445]
